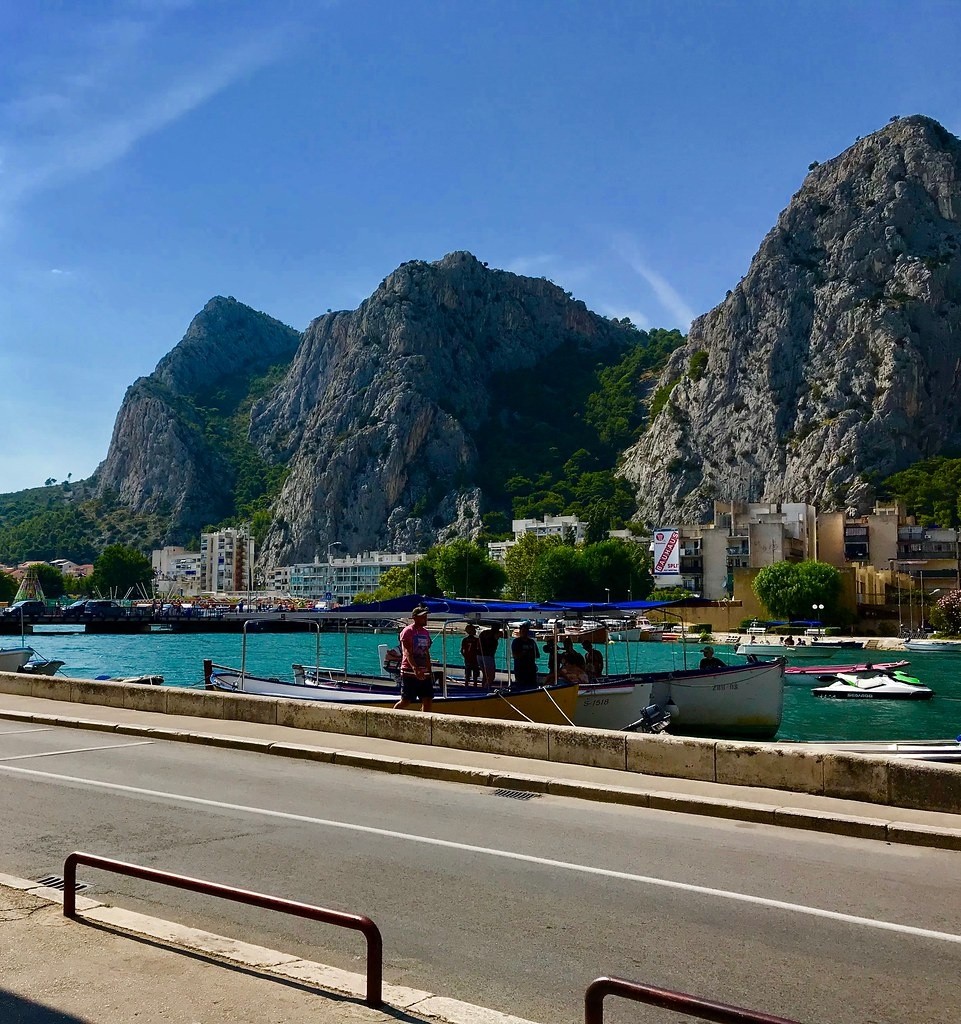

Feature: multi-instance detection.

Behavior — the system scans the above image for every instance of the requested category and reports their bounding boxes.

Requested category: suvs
[3,600,46,619]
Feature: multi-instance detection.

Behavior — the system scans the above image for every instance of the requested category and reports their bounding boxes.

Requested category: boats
[211,665,582,727]
[94,675,164,685]
[513,618,665,643]
[810,640,864,650]
[304,655,653,729]
[430,656,786,739]
[809,669,934,702]
[735,642,842,658]
[0,645,65,676]
[903,642,961,651]
[744,655,912,685]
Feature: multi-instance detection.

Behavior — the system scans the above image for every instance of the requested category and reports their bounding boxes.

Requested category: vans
[84,600,127,618]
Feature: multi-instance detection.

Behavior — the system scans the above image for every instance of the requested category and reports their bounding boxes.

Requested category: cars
[61,599,88,619]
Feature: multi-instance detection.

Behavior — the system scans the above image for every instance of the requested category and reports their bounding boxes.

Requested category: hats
[563,638,573,648]
[581,640,592,648]
[700,646,714,653]
[412,607,431,616]
[466,625,479,633]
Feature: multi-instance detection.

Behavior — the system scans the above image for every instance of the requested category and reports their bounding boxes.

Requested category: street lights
[328,542,342,591]
[812,603,824,637]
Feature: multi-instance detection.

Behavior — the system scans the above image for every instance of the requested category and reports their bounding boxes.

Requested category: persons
[386,631,444,696]
[55,600,62,617]
[511,624,540,684]
[476,623,508,688]
[390,606,435,712]
[750,634,818,646]
[543,637,603,679]
[38,598,46,607]
[149,593,333,619]
[461,625,480,687]
[699,646,728,670]
